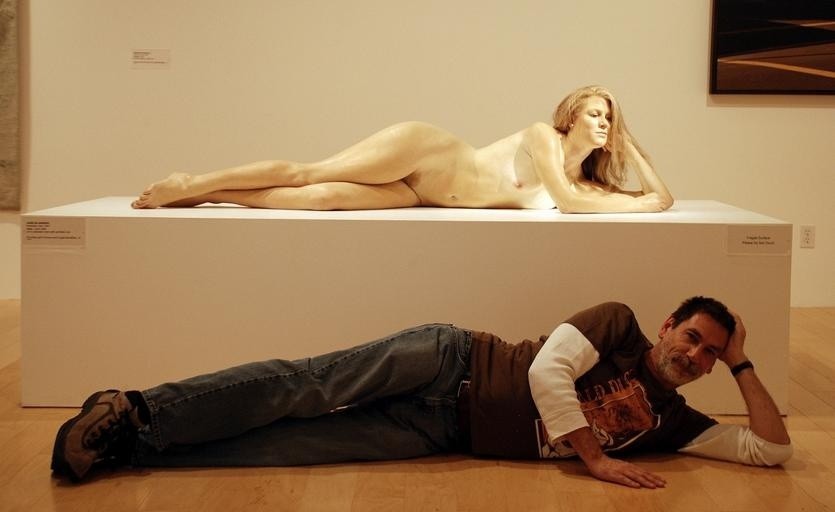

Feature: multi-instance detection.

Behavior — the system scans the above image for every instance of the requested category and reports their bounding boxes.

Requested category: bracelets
[729,360,754,376]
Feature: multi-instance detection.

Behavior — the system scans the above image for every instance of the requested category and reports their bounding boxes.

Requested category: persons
[126,84,676,216]
[49,294,792,492]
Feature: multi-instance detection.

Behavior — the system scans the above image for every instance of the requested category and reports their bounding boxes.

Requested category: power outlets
[800,224,815,249]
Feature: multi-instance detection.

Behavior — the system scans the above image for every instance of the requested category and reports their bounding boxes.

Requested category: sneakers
[49,389,152,480]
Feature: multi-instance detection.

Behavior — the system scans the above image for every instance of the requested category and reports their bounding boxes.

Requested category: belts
[453,365,473,457]
